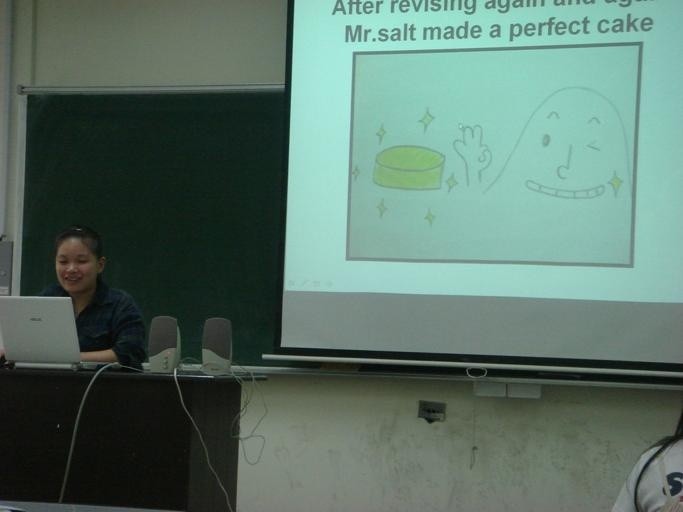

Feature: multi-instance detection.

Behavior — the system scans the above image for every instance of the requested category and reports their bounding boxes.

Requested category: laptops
[0,294,122,373]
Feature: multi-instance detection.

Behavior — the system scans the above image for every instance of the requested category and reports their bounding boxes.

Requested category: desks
[0,362,244,511]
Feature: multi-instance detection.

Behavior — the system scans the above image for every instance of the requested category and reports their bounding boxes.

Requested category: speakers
[149,316,181,373]
[203,318,232,375]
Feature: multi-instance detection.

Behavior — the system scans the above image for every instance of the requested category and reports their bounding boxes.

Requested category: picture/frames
[261,4,681,378]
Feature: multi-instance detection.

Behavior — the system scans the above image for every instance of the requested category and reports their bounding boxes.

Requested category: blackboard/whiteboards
[15,86,683,390]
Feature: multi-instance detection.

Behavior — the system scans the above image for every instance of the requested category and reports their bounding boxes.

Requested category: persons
[0,226,147,367]
[609,408,682,512]
